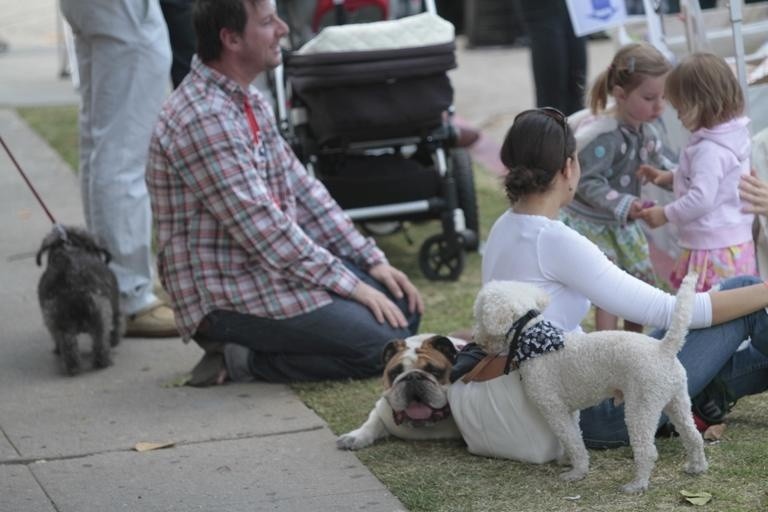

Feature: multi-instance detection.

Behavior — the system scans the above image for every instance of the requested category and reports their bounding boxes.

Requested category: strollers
[264,1,483,285]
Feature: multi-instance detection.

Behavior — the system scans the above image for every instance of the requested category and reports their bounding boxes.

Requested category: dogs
[334,331,471,452]
[35,225,123,376]
[470,268,709,495]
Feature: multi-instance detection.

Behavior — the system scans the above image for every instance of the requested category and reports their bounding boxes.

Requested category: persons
[526,0,588,117]
[441,105,480,147]
[482,106,767,449]
[736,167,768,238]
[551,42,674,335]
[59,0,182,337]
[634,52,761,296]
[143,0,424,387]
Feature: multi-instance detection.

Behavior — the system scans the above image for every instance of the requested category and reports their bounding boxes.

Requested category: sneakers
[123,286,181,339]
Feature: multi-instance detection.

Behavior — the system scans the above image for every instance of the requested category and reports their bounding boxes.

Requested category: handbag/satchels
[447,351,562,465]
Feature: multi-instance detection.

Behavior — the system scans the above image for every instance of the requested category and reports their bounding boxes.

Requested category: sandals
[187,339,228,387]
